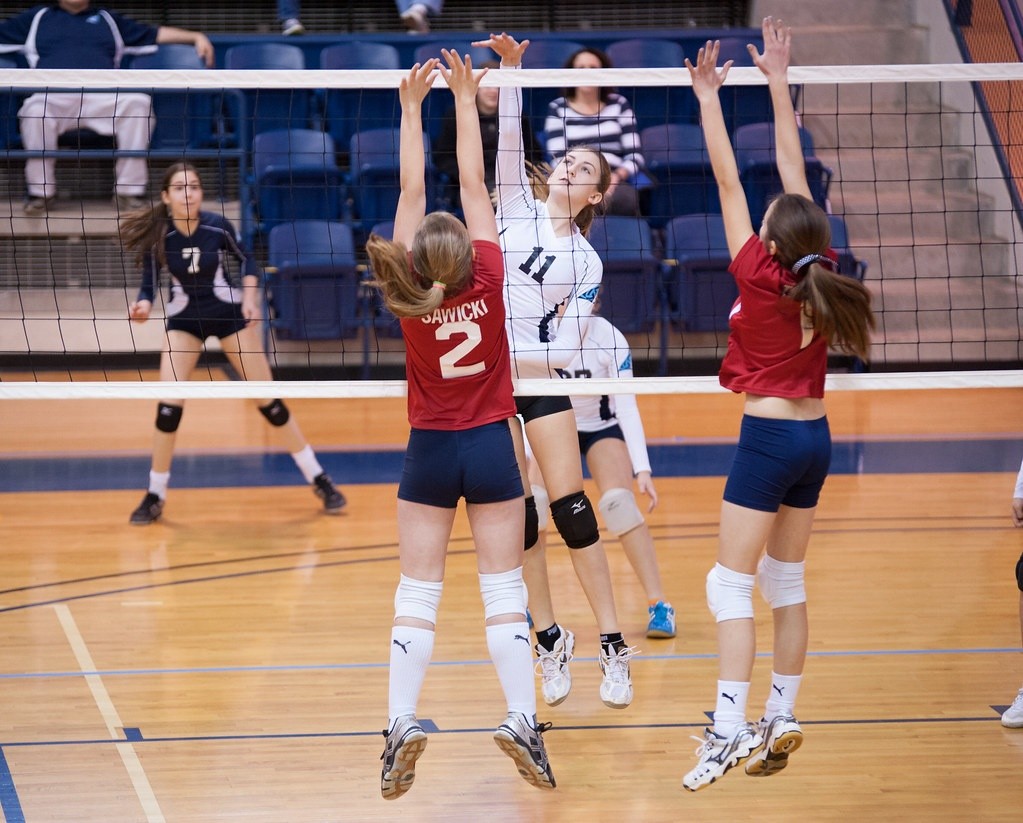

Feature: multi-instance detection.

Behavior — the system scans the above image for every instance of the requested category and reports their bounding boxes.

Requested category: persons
[394,0,444,35]
[431,62,545,225]
[0,0,214,217]
[1001,459,1023,729]
[524,296,678,640]
[278,0,305,36]
[470,29,632,711]
[683,15,878,795]
[118,161,348,525]
[545,49,646,216]
[366,49,556,800]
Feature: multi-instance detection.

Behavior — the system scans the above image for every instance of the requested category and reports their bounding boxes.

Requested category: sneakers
[682,722,763,792]
[1001,689,1023,727]
[646,600,677,637]
[129,494,164,524]
[598,644,642,709]
[494,712,556,791]
[534,625,575,706]
[312,471,349,512]
[746,715,804,777]
[380,714,427,800]
[526,607,534,629]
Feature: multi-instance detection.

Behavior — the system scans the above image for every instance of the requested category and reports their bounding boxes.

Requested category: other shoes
[24,195,57,214]
[283,17,304,34]
[400,5,432,36]
[113,194,147,210]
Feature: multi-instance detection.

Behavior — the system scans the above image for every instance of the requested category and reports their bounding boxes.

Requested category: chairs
[0,30,868,375]
